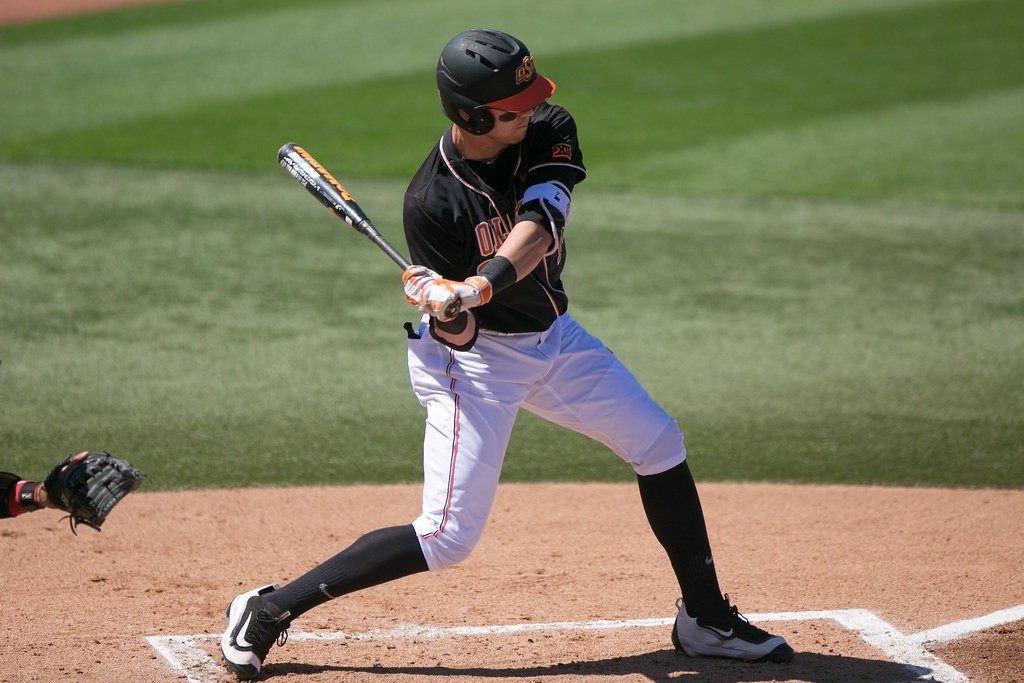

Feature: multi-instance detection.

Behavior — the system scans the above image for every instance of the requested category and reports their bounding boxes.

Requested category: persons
[0,451,143,536]
[219,30,793,676]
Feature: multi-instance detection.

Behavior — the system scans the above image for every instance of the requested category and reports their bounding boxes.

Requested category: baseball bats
[275,141,460,319]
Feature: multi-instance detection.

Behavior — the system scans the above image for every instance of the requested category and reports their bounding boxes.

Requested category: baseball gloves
[44,452,143,530]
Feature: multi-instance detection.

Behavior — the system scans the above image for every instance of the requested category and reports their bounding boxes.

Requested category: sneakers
[220,584,291,679]
[671,593,794,664]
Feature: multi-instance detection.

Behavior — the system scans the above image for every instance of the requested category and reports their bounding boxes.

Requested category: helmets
[435,29,555,136]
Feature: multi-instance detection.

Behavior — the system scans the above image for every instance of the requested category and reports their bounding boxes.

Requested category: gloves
[418,274,492,322]
[401,266,444,308]
